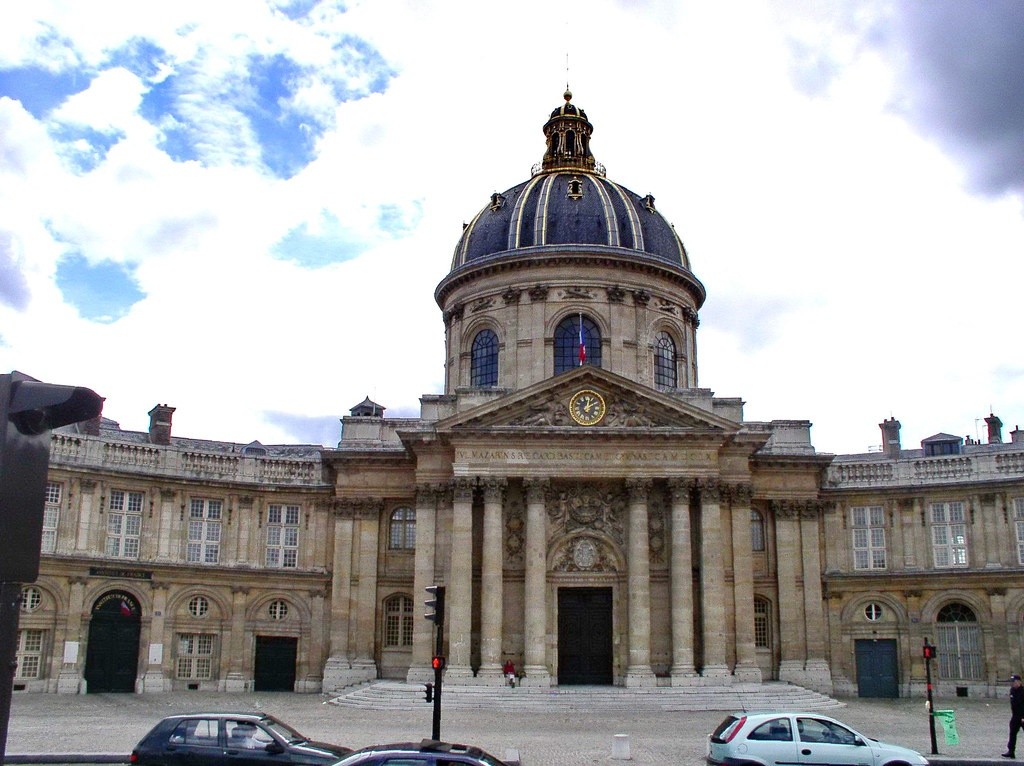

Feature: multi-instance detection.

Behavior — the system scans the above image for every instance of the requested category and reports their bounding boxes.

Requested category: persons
[607,394,657,427]
[503,659,516,688]
[519,395,566,426]
[1002,675,1024,759]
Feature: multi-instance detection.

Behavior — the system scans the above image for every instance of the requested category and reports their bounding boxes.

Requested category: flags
[121,601,131,616]
[577,319,587,363]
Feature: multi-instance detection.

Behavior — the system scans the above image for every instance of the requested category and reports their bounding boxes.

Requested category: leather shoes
[1001,750,1016,758]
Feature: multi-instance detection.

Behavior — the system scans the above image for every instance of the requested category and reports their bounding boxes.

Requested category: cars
[327,741,518,766]
[123,713,355,766]
[705,709,930,766]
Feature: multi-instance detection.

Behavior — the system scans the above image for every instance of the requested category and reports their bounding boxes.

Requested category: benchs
[174,727,199,746]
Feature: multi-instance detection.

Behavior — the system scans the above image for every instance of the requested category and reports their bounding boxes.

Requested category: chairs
[216,727,244,747]
[772,724,787,740]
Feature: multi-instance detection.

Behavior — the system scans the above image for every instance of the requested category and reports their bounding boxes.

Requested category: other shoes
[509,678,513,684]
[511,682,515,688]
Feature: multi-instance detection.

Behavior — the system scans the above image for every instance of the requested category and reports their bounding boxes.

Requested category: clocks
[569,390,606,426]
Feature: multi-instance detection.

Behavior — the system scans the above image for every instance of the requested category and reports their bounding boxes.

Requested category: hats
[1008,674,1022,682]
